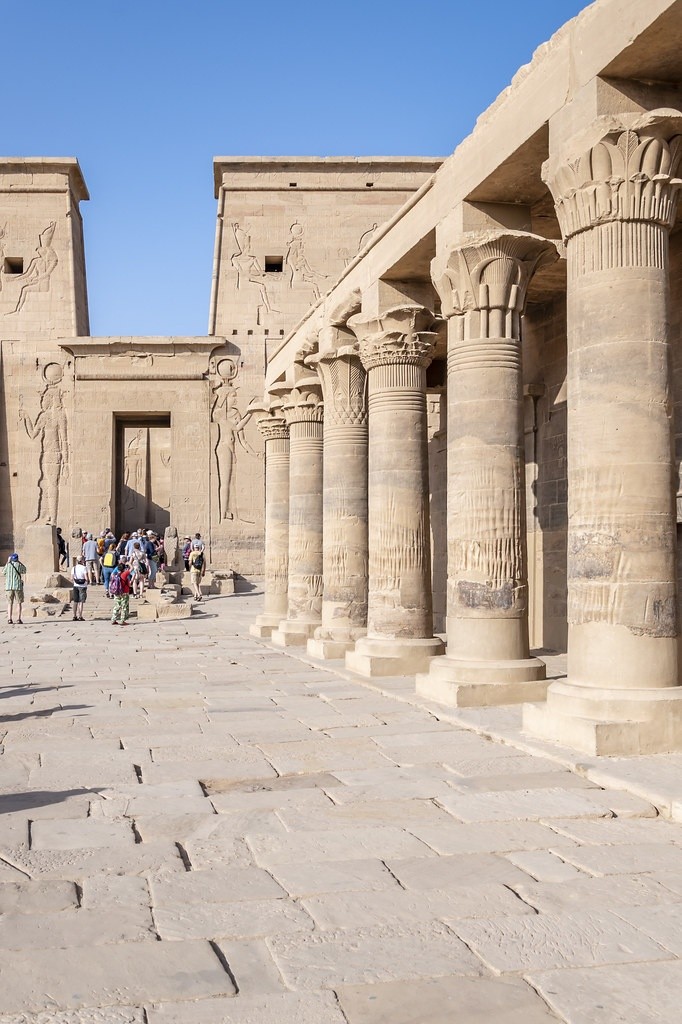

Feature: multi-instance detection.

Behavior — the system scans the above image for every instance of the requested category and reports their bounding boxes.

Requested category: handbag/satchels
[74,578,86,584]
[183,547,191,558]
[139,562,147,574]
[192,552,203,570]
[152,555,158,561]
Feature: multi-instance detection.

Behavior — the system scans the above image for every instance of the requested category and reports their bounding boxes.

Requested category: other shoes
[79,617,85,621]
[194,595,202,601]
[120,621,129,625]
[111,621,118,625]
[73,616,78,621]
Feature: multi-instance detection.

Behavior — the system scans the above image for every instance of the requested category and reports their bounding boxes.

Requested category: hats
[100,531,106,537]
[77,555,86,562]
[184,536,191,541]
[86,533,92,538]
[132,532,138,537]
[11,554,18,561]
[194,544,199,547]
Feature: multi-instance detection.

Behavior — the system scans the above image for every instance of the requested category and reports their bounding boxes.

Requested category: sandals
[17,619,23,624]
[8,619,13,624]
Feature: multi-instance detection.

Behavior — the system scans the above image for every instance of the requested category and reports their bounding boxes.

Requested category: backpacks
[104,551,116,566]
[110,572,127,594]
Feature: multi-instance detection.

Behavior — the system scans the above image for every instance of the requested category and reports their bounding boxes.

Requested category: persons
[181,535,192,572]
[71,555,88,621]
[82,533,101,585]
[56,527,68,570]
[99,543,120,598]
[111,556,136,584]
[2,553,26,624]
[111,562,134,625]
[81,530,87,543]
[128,542,146,599]
[97,527,165,589]
[190,533,206,553]
[188,544,206,601]
